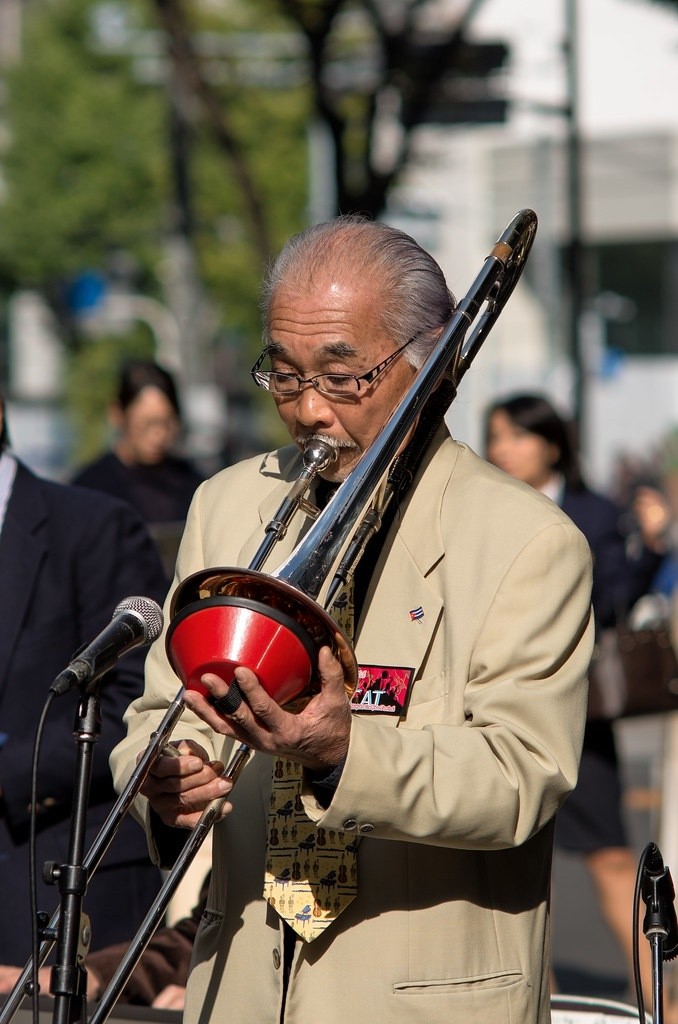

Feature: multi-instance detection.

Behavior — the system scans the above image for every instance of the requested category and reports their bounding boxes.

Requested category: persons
[623,468,678,603]
[107,213,596,1024]
[0,869,215,1011]
[484,395,678,1024]
[71,358,205,583]
[0,400,191,968]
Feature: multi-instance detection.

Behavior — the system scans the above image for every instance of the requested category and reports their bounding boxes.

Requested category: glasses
[250,329,424,397]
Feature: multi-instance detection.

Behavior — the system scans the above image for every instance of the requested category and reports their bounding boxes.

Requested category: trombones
[2,210,539,1022]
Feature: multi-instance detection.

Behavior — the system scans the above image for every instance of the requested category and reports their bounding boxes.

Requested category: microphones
[49,596,164,696]
[642,844,678,961]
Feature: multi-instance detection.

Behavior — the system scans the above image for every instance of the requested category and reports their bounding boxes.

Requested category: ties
[263,571,356,943]
